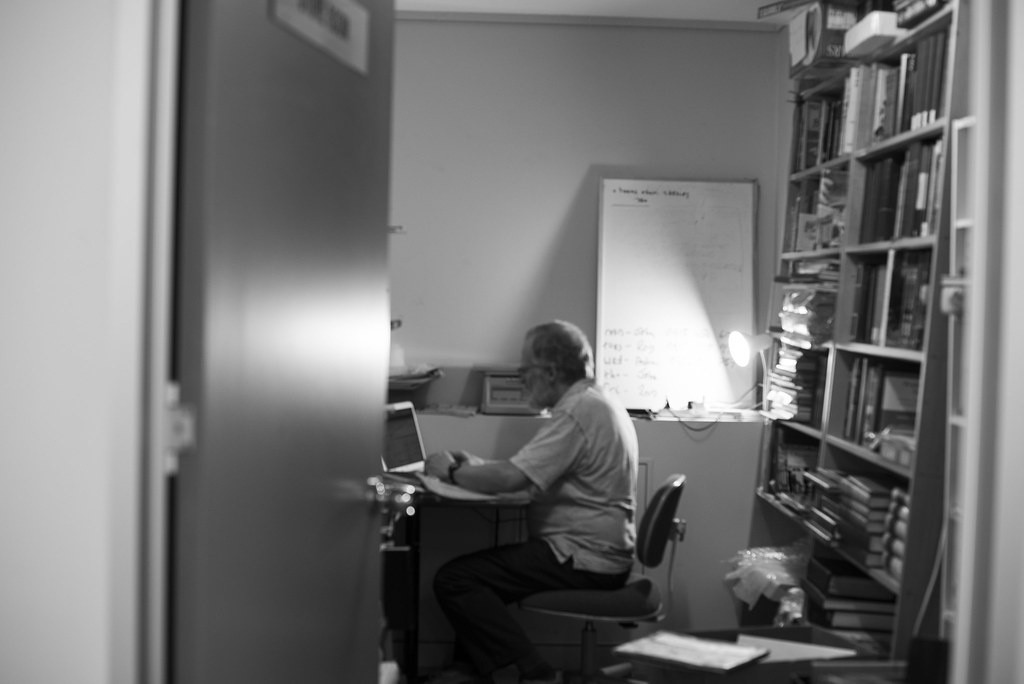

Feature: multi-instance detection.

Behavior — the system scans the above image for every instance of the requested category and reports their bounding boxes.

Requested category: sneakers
[520,669,564,684]
[428,669,474,684]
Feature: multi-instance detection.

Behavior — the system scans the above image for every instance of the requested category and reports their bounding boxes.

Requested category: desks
[381,470,540,683]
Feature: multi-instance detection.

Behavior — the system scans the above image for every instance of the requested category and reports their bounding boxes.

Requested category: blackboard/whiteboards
[594,177,761,414]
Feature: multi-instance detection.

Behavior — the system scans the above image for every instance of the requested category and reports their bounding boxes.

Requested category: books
[767,0,948,632]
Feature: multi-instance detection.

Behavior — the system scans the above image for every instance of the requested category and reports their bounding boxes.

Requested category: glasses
[517,365,538,374]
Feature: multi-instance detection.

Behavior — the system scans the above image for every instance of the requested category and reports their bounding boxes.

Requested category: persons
[425,320,638,684]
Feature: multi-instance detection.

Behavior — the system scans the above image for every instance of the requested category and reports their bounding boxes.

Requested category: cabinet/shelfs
[734,0,967,662]
[941,110,974,683]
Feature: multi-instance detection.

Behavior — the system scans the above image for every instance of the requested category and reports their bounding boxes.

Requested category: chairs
[515,471,690,684]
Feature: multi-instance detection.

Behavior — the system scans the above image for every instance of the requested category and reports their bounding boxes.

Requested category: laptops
[379,401,427,474]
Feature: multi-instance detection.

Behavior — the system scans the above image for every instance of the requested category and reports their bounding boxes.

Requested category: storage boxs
[684,621,870,683]
[788,0,860,81]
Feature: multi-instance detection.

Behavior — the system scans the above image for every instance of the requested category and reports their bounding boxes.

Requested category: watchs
[449,462,460,484]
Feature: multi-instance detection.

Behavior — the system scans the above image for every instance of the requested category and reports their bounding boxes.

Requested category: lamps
[726,329,774,417]
